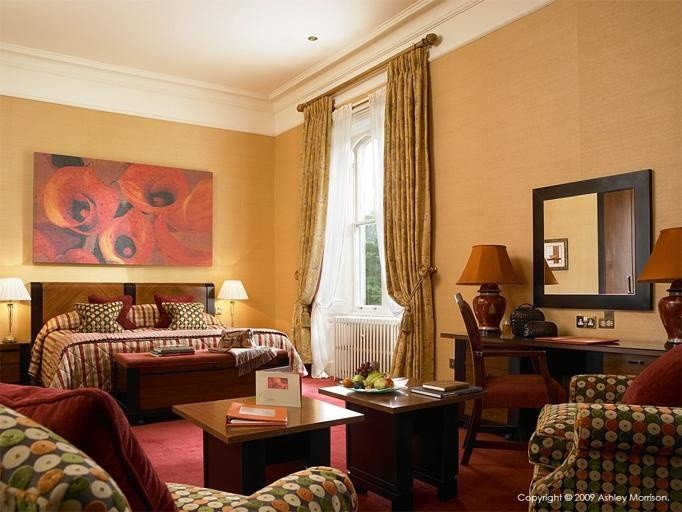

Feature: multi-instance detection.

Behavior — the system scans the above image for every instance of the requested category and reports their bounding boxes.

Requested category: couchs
[529,346,682,512]
[0,384,359,512]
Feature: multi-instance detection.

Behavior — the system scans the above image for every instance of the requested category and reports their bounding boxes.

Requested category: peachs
[385,378,394,387]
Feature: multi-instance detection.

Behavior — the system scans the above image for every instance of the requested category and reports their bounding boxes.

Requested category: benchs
[111,346,288,427]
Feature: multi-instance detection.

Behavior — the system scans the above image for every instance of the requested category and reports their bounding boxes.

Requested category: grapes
[352,381,364,391]
[355,362,372,377]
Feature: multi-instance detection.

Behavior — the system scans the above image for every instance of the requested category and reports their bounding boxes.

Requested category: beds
[30,282,293,421]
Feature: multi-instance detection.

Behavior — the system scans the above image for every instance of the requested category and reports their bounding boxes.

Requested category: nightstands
[1,344,32,384]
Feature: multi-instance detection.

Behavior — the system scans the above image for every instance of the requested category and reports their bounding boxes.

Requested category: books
[224,399,288,428]
[149,343,196,359]
[409,377,485,399]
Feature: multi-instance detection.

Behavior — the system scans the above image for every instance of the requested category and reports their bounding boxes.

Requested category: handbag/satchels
[511,303,557,338]
[221,329,257,348]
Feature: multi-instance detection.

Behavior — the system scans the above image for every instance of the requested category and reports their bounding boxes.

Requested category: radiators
[334,316,407,380]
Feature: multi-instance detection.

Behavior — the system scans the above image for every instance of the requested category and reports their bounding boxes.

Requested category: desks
[440,330,675,428]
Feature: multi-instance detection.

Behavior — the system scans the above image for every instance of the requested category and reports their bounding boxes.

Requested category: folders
[225,401,288,426]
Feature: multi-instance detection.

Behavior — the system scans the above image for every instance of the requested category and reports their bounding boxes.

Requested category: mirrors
[533,169,653,310]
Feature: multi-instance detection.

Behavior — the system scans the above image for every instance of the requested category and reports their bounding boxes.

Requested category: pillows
[73,293,208,334]
[621,344,682,406]
[1,384,178,512]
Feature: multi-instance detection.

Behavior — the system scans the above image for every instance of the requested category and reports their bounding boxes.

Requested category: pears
[352,374,365,385]
[374,378,386,390]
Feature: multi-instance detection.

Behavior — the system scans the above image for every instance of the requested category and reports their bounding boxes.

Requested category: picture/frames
[544,238,568,270]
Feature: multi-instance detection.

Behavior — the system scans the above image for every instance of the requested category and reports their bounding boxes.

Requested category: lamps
[0,278,31,343]
[217,280,248,329]
[636,228,682,349]
[456,244,522,338]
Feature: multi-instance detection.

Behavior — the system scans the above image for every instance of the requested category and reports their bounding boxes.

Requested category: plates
[338,377,411,393]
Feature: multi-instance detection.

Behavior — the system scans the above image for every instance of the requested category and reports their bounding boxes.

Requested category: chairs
[455,292,567,465]
